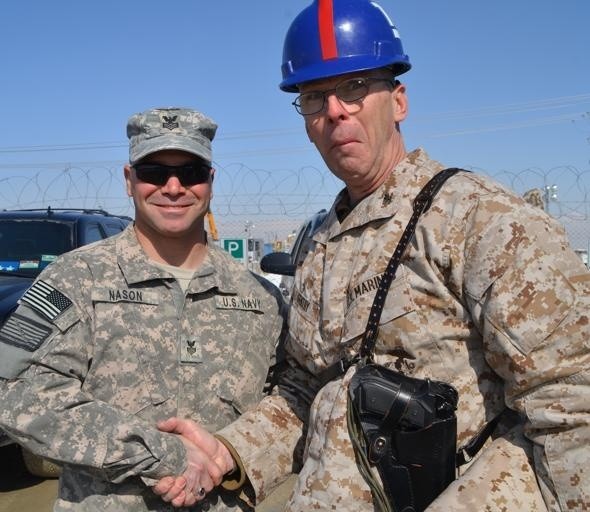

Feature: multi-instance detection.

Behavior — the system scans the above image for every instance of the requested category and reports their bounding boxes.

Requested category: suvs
[1,208,136,485]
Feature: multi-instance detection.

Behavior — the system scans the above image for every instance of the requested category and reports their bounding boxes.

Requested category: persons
[151,0,590,512]
[0,106,289,512]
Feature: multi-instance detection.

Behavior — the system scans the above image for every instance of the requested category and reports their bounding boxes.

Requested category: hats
[126,108,218,171]
[279,1,412,93]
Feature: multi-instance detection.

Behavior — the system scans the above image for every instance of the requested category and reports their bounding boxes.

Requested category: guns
[356,376,457,512]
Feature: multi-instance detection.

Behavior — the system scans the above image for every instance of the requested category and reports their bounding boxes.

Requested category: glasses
[292,76,393,117]
[132,160,210,187]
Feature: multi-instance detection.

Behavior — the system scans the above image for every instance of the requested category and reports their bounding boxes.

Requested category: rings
[192,481,206,498]
